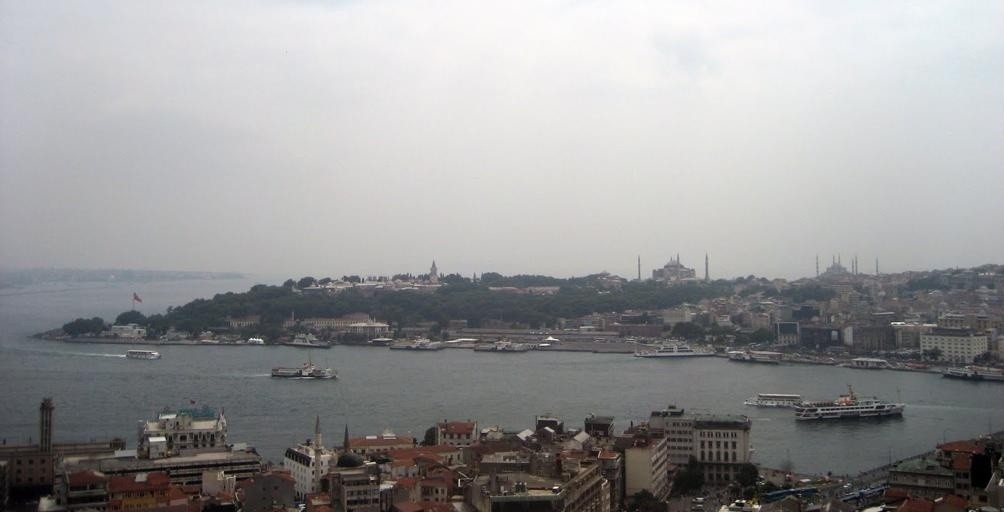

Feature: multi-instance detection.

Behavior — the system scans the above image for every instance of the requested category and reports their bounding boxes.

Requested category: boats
[728,350,783,363]
[247,336,265,345]
[943,364,1004,381]
[633,337,715,357]
[744,384,906,421]
[125,350,160,360]
[851,357,888,369]
[406,335,531,352]
[271,330,336,380]
